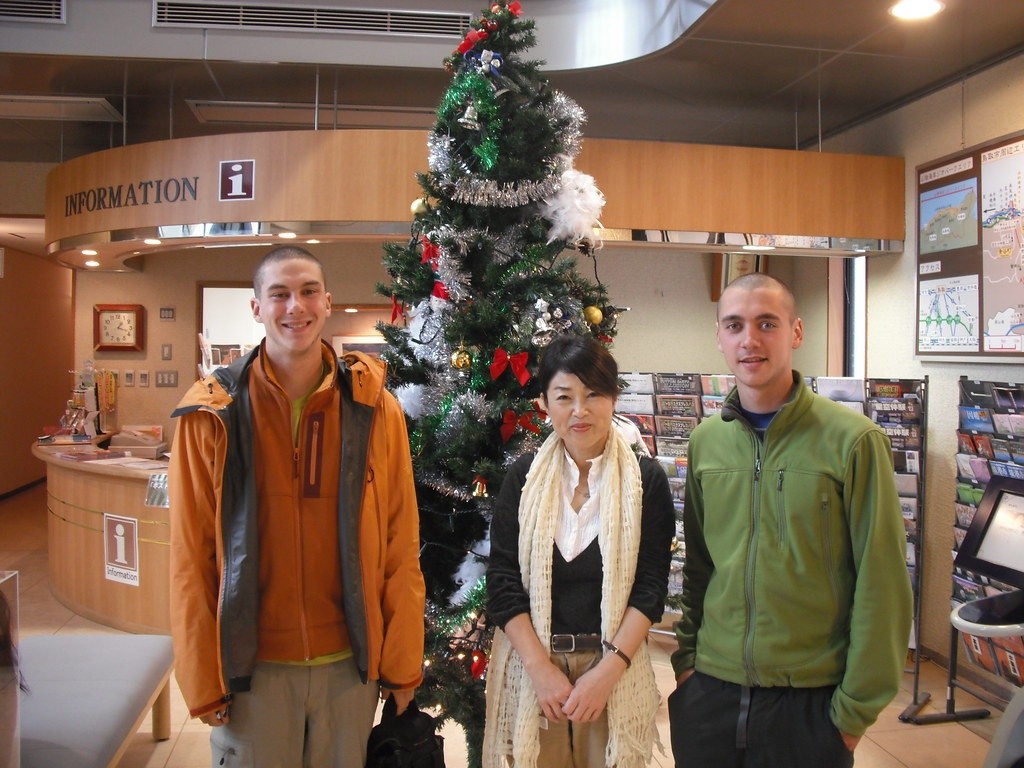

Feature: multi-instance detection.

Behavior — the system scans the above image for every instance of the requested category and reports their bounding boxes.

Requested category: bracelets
[602,640,631,669]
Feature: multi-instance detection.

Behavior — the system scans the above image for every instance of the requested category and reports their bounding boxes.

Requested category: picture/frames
[711,252,770,304]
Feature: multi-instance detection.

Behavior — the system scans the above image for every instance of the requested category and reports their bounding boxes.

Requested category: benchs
[16,634,175,768]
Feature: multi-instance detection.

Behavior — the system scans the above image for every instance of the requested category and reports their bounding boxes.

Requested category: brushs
[37,435,52,444]
[72,434,92,441]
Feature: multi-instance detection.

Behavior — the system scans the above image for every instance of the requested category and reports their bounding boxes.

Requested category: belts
[550,634,603,654]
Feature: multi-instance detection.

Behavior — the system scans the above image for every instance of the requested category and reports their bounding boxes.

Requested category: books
[614,374,921,649]
[61,449,131,462]
[951,406,1024,687]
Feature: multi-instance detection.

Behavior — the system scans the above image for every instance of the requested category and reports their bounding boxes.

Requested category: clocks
[92,303,143,351]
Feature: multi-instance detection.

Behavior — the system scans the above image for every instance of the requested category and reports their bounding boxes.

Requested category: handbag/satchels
[365,692,446,768]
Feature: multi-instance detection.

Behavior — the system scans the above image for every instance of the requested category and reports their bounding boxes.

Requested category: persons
[481,336,677,768]
[667,272,913,768]
[167,246,427,768]
